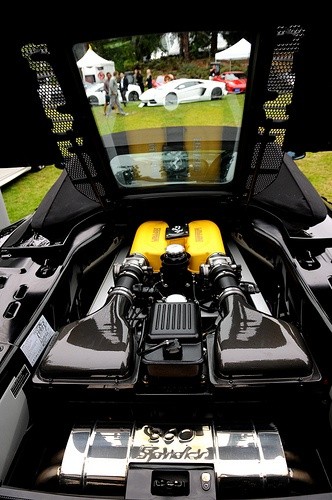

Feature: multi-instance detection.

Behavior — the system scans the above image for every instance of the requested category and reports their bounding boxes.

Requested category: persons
[209,63,218,82]
[98,67,172,118]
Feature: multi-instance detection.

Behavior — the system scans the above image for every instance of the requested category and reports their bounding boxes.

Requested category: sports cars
[213,70,248,94]
[86,83,142,106]
[139,78,228,107]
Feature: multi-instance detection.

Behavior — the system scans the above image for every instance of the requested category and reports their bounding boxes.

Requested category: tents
[76,44,115,81]
[214,37,251,72]
